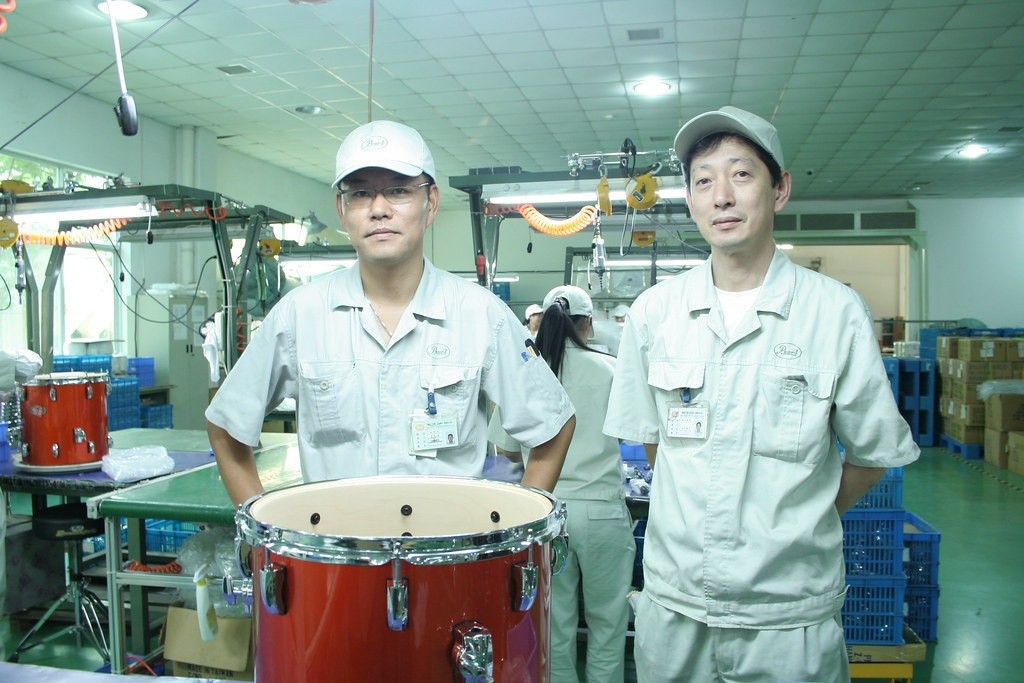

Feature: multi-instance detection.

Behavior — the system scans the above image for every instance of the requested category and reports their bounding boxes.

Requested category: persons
[483,282,638,683]
[521,303,630,356]
[601,103,922,682]
[202,118,577,512]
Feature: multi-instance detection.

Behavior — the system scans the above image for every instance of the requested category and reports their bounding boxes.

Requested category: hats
[674,105,783,187]
[525,304,543,320]
[543,284,593,317]
[610,304,630,318]
[331,120,437,187]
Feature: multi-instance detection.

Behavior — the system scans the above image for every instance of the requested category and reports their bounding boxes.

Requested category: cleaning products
[193,563,219,641]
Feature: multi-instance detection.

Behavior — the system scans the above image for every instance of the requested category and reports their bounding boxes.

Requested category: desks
[0,428,304,683]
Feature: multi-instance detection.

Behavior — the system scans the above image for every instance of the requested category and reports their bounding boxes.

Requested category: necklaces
[365,293,393,337]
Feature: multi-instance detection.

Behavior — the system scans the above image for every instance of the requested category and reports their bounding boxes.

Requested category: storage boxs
[52,353,181,427]
[163,599,257,683]
[92,527,129,555]
[833,318,1024,683]
[146,517,207,553]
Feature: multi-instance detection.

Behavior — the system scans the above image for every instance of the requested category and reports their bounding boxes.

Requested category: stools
[7,502,130,666]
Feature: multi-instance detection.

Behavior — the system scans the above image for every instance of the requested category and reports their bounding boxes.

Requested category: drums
[232,470,570,683]
[15,371,110,473]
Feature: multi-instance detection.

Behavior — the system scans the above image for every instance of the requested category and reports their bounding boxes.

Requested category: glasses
[334,183,431,209]
